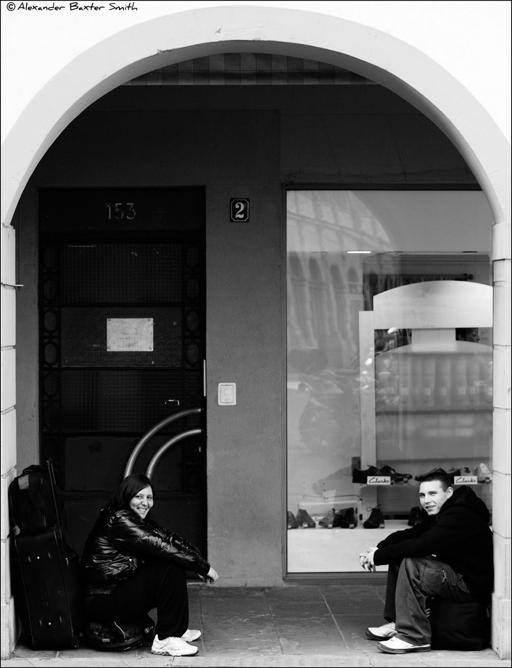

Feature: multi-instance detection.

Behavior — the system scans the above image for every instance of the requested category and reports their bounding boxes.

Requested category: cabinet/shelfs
[287,519,415,574]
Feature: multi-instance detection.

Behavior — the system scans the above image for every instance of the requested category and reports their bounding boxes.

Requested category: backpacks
[86,612,155,653]
[426,595,490,651]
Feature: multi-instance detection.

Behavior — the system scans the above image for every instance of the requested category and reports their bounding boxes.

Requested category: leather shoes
[349,463,492,484]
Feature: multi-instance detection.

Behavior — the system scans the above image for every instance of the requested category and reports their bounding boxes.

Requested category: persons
[79,472,218,658]
[360,472,492,655]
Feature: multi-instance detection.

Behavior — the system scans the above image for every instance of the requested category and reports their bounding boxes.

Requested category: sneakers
[180,629,202,641]
[150,633,199,657]
[365,622,397,640]
[364,505,385,529]
[287,504,357,530]
[376,635,433,653]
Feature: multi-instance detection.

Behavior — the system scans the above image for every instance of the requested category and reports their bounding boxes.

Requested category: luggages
[10,460,83,646]
